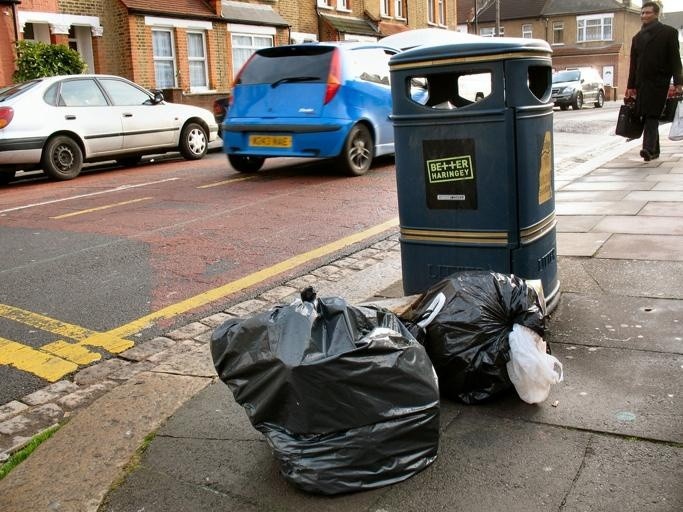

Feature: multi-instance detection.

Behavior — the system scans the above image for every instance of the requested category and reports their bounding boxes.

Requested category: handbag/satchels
[668,93,683,142]
[614,96,645,140]
[658,95,683,126]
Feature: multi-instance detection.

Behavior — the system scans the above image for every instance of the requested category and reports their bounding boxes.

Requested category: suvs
[550,65,607,109]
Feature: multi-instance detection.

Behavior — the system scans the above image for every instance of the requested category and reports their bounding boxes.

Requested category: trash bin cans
[389,37,566,317]
[161,88,183,104]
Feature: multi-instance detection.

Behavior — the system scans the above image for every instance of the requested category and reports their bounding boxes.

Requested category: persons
[625,2,683,161]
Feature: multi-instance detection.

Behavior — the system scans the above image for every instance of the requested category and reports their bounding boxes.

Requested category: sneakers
[639,149,660,162]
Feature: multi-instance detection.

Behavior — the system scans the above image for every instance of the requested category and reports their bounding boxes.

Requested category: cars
[667,76,683,100]
